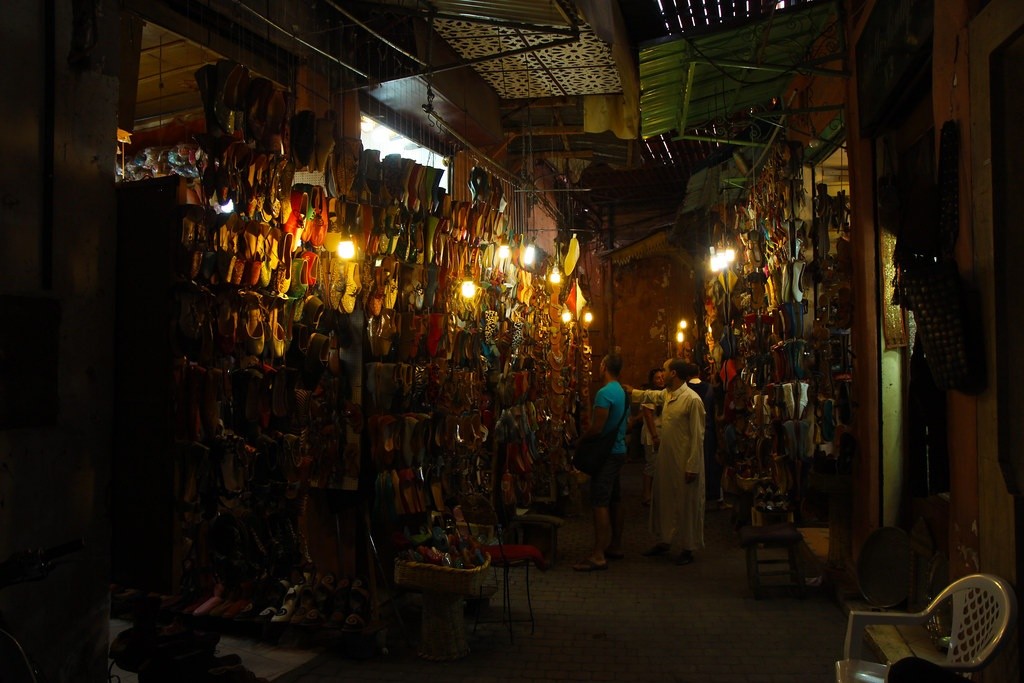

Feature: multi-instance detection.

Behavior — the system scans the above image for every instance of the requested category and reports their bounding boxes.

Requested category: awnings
[636,0,852,148]
[381,0,624,100]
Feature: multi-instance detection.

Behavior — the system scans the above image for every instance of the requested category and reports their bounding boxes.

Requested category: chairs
[834,571,1018,683]
[460,492,541,647]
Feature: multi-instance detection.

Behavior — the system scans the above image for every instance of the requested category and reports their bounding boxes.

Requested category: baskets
[420,593,470,662]
[395,549,492,596]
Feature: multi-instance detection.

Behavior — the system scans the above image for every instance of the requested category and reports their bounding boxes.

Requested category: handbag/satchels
[576,429,617,468]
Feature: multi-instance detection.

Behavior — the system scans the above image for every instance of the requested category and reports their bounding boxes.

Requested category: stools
[514,514,564,569]
[740,521,807,598]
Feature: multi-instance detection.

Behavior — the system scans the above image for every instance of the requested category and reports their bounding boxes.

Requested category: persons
[573,353,716,573]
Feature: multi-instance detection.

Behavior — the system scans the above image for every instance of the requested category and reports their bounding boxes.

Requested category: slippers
[572,557,607,571]
[604,547,622,560]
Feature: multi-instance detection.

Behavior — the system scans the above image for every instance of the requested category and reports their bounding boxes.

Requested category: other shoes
[113,61,593,640]
[676,549,695,564]
[643,543,671,559]
[692,136,853,525]
